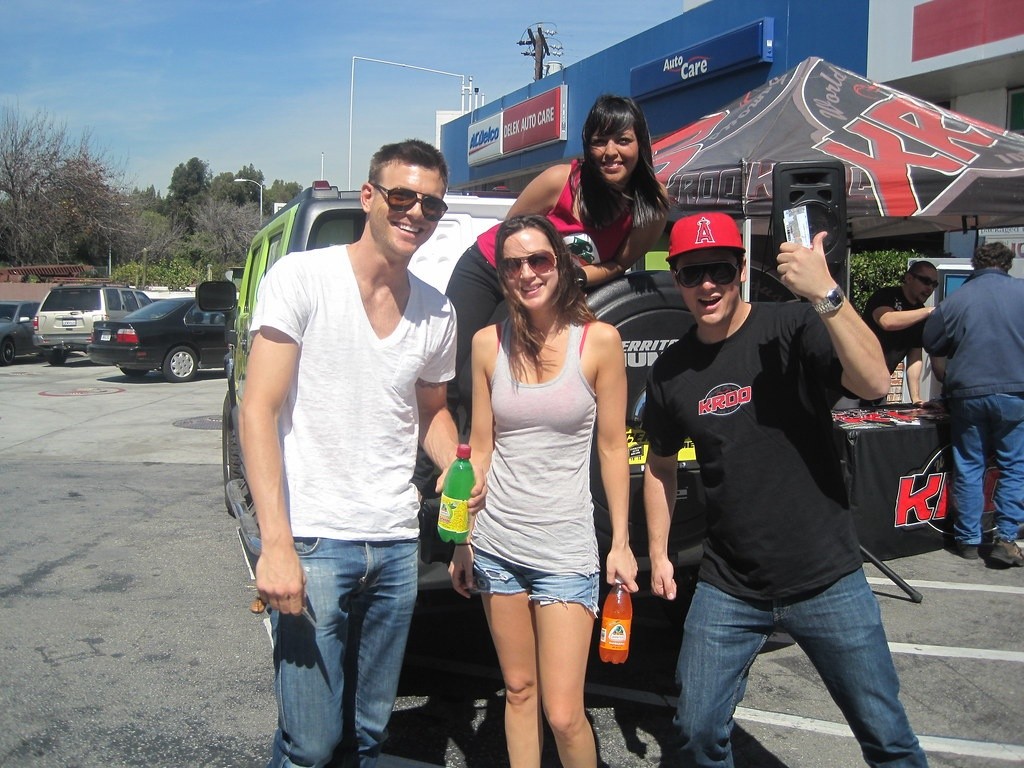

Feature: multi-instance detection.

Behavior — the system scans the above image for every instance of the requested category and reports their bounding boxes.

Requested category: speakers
[772,160,847,264]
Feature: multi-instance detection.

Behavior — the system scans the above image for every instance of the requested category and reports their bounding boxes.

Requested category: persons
[409,94,669,502]
[449,214,639,768]
[860,261,939,407]
[923,242,1024,566]
[641,212,929,768]
[237,139,488,768]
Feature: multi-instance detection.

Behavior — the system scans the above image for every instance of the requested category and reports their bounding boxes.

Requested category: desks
[832,402,997,563]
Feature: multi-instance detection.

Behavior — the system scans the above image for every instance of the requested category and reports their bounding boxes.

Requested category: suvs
[221,180,706,660]
[32,284,154,367]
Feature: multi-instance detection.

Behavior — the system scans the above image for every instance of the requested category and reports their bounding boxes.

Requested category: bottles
[437,444,476,544]
[599,575,632,664]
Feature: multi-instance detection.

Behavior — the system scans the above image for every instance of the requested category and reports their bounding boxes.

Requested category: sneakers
[989,537,1024,567]
[956,542,979,559]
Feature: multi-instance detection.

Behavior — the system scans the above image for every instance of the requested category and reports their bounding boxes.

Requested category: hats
[666,212,746,262]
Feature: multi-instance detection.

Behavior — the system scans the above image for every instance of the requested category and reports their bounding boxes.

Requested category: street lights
[234,178,262,227]
[108,203,130,276]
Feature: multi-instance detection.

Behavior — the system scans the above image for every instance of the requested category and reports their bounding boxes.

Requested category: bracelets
[455,543,471,546]
[454,543,471,546]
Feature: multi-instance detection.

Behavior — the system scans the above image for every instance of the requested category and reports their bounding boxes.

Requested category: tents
[651,56,1024,303]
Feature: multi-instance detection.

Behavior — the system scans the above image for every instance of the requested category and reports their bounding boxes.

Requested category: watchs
[812,283,844,315]
[812,284,845,315]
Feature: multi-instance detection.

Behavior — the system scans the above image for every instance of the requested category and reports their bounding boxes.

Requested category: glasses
[499,251,557,279]
[377,184,448,222]
[911,272,939,288]
[674,261,739,288]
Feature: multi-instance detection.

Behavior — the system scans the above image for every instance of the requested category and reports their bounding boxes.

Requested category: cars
[86,297,228,383]
[0,301,41,367]
